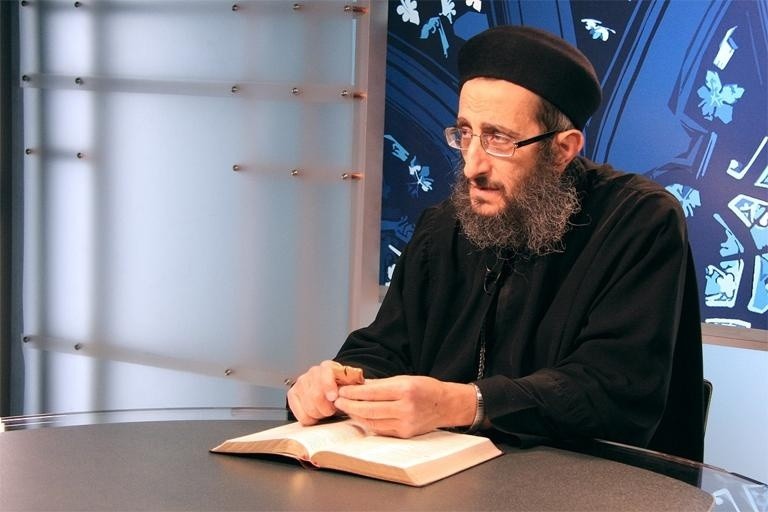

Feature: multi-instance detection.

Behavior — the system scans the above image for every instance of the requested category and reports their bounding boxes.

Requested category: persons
[284,29,712,491]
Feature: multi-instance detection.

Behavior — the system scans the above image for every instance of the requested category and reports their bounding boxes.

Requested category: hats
[459,25,602,132]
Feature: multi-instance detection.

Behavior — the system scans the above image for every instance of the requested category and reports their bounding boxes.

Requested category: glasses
[442,124,556,159]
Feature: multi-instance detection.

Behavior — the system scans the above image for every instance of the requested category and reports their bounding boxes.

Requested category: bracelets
[461,382,486,436]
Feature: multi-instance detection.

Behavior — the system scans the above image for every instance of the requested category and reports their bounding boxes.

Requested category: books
[209,409,505,488]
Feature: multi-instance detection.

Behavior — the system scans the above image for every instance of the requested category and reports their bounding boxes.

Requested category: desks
[0,406,768,512]
